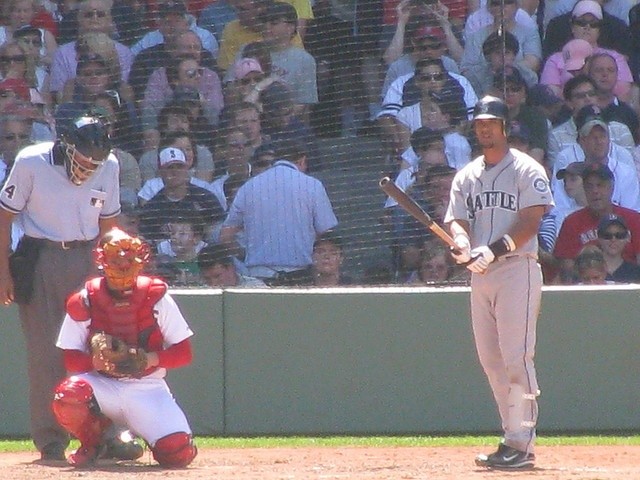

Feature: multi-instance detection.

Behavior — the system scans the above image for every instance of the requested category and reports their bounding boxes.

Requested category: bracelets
[488,234,516,257]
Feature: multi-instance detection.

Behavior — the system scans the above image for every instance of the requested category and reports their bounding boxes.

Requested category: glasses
[576,254,602,261]
[0,134,29,139]
[597,231,628,240]
[573,90,597,97]
[83,72,104,76]
[185,68,204,80]
[84,11,104,17]
[254,159,277,165]
[102,90,121,108]
[491,1,514,6]
[21,38,41,46]
[499,84,523,92]
[241,74,266,84]
[415,43,442,50]
[0,55,26,61]
[421,74,443,81]
[0,92,21,98]
[228,142,250,148]
[572,18,602,28]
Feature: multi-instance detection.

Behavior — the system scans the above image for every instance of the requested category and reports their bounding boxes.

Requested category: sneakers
[68,445,108,467]
[42,442,65,459]
[97,437,143,459]
[475,443,504,467]
[487,448,535,469]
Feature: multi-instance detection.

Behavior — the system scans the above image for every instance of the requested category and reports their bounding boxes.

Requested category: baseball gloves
[91,333,148,374]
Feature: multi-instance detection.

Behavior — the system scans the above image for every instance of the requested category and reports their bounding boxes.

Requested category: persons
[443,95,556,469]
[490,1,539,162]
[0,1,73,193]
[53,227,197,468]
[143,1,490,289]
[540,1,640,286]
[0,117,143,461]
[103,117,143,227]
[74,1,143,116]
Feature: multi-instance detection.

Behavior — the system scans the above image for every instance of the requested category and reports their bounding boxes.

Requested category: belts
[22,234,94,251]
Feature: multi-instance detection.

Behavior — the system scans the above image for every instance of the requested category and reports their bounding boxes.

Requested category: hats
[234,57,265,79]
[263,80,293,116]
[0,77,31,100]
[432,90,467,119]
[411,127,455,153]
[159,2,186,17]
[555,161,588,179]
[76,53,104,74]
[313,227,343,250]
[159,147,187,169]
[483,30,518,56]
[259,2,297,22]
[576,104,608,136]
[0,104,33,121]
[561,39,593,70]
[254,144,276,155]
[13,23,43,37]
[599,214,627,230]
[172,83,199,105]
[275,140,310,158]
[582,163,614,181]
[413,24,444,38]
[527,84,563,106]
[571,1,603,18]
[494,66,520,83]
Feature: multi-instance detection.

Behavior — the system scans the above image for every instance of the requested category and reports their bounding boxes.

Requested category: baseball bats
[380,178,458,254]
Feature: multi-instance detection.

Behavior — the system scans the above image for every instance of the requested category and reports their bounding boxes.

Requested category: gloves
[467,245,495,272]
[451,235,470,265]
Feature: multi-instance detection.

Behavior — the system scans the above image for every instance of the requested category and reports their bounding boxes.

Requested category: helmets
[97,226,151,290]
[64,113,110,186]
[470,95,511,137]
